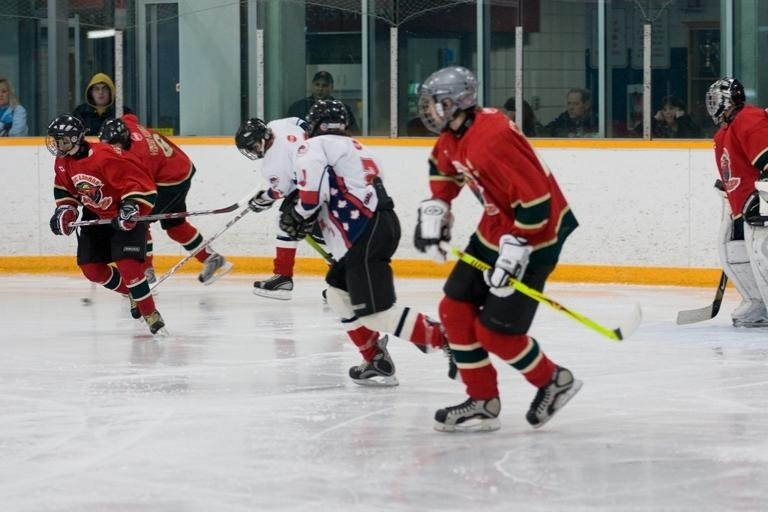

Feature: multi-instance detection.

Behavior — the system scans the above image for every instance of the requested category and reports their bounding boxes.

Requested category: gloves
[413,198,456,262]
[481,232,532,298]
[248,187,276,214]
[50,204,79,236]
[111,201,141,232]
[278,199,322,241]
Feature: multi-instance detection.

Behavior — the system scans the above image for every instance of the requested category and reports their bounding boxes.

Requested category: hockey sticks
[66,187,259,228]
[439,240,642,341]
[677,272,728,326]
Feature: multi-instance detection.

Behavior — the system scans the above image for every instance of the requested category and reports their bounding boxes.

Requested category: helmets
[304,98,350,138]
[97,117,131,150]
[235,118,271,161]
[705,76,746,125]
[418,66,477,133]
[44,113,86,158]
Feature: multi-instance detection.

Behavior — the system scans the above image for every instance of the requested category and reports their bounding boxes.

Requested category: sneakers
[349,335,396,380]
[424,313,458,380]
[253,273,294,291]
[127,292,141,319]
[142,310,165,334]
[525,366,575,425]
[198,253,225,283]
[433,395,501,425]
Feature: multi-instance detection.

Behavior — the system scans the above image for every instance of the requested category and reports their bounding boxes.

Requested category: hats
[312,70,332,82]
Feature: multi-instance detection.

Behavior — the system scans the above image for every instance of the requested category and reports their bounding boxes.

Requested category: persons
[288,71,360,136]
[634,97,702,139]
[414,64,583,432]
[0,78,29,136]
[543,87,599,137]
[235,116,337,301]
[45,115,169,339]
[291,98,463,388]
[70,72,131,136]
[97,114,233,288]
[504,96,542,137]
[705,77,768,328]
